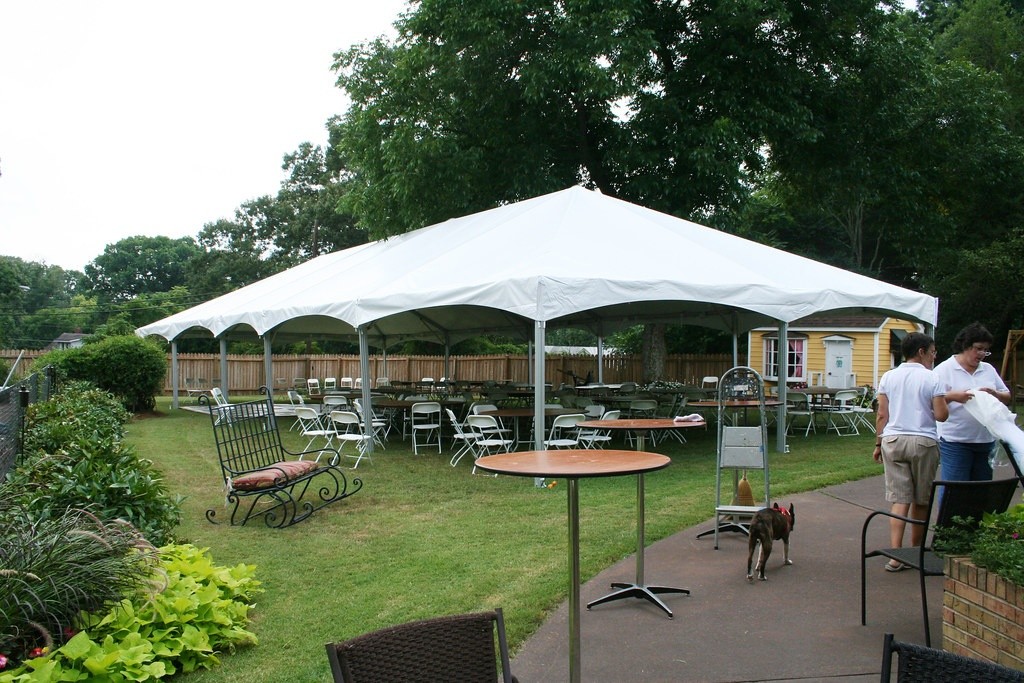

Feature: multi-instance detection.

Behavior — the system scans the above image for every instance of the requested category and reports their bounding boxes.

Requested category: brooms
[729,469,755,506]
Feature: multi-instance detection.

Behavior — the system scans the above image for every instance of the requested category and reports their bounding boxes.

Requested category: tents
[135,185,938,489]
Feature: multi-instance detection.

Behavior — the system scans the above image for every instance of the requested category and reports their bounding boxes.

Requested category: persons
[933,323,1011,528]
[872,332,949,571]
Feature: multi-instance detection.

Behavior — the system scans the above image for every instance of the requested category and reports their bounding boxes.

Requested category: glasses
[925,351,937,356]
[974,347,991,356]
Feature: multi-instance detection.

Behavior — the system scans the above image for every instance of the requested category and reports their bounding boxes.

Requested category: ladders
[713,366,771,551]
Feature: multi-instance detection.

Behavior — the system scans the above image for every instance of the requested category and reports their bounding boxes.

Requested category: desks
[474,449,673,683]
[309,393,362,415]
[786,387,868,429]
[652,386,720,394]
[479,408,590,446]
[370,400,468,449]
[481,388,535,407]
[575,418,707,620]
[572,383,639,397]
[685,400,785,544]
[683,400,784,416]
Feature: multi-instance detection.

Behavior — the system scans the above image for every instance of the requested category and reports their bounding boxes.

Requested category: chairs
[322,606,523,683]
[875,630,1024,683]
[861,473,1020,644]
[180,375,880,479]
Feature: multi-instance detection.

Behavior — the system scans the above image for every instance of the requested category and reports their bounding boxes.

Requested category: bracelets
[875,443,881,447]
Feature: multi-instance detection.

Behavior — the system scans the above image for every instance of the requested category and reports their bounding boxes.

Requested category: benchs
[197,385,364,531]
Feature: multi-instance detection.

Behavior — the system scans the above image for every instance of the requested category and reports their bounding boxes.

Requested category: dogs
[745,502,795,581]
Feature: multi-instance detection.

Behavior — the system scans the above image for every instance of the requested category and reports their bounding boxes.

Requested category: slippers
[885,561,912,572]
